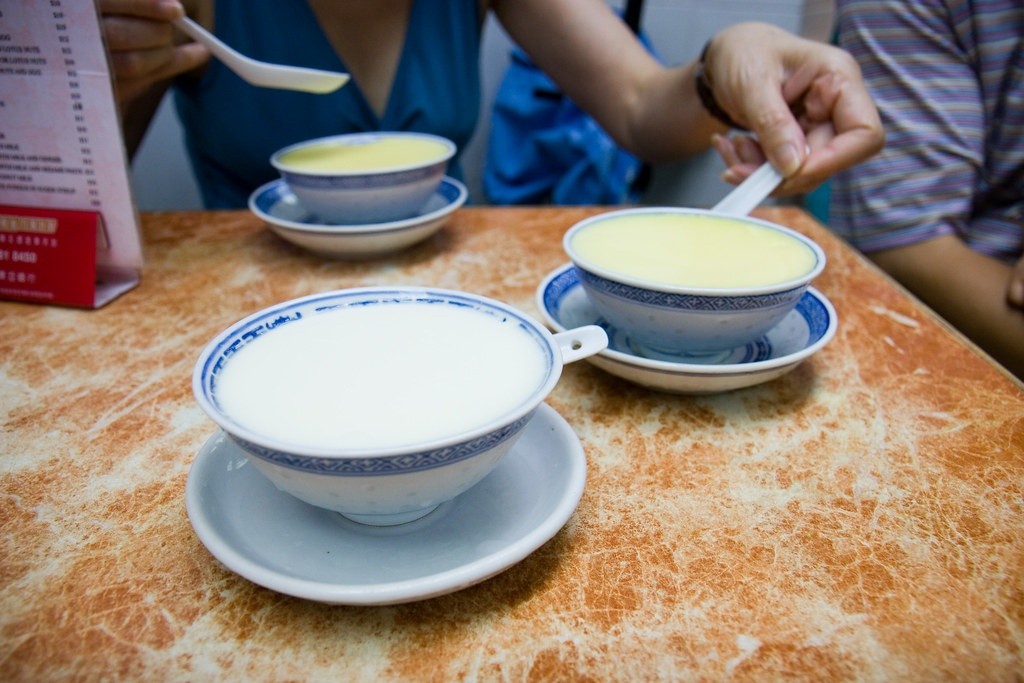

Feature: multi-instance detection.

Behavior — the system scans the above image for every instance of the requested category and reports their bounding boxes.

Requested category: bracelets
[694,44,749,132]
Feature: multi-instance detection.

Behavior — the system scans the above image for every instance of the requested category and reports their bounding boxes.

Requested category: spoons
[173,16,350,93]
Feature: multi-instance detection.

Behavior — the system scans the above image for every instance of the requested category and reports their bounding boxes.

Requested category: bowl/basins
[191,284,567,527]
[269,131,457,224]
[248,177,469,259]
[561,206,826,359]
[536,262,838,396]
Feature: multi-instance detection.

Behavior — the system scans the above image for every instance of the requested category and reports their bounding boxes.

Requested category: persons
[828,0,1024,384]
[92,0,884,211]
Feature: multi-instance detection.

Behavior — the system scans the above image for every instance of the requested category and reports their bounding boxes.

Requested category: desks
[0,204,1024,683]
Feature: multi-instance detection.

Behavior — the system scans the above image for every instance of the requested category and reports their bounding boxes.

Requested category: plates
[184,400,587,605]
